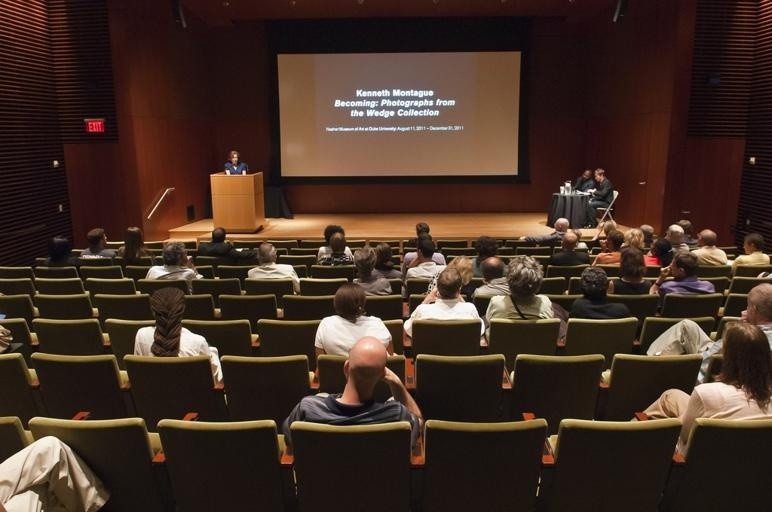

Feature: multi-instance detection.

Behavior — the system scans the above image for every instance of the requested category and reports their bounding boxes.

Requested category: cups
[564,180,571,195]
[559,186,565,194]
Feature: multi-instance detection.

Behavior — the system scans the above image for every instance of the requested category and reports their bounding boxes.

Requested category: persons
[0,324,13,354]
[584,167,613,229]
[574,168,596,191]
[281,336,424,450]
[630,321,772,457]
[224,149,248,176]
[0,436,119,512]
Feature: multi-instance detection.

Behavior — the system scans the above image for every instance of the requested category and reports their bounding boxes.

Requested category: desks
[546,190,595,230]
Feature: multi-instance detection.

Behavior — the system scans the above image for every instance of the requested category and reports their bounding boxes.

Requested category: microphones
[225,163,235,174]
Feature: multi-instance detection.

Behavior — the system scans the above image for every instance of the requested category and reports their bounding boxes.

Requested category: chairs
[0,235,771,512]
[596,190,619,226]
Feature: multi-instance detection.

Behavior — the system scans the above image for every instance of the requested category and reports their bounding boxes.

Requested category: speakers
[703,73,720,85]
